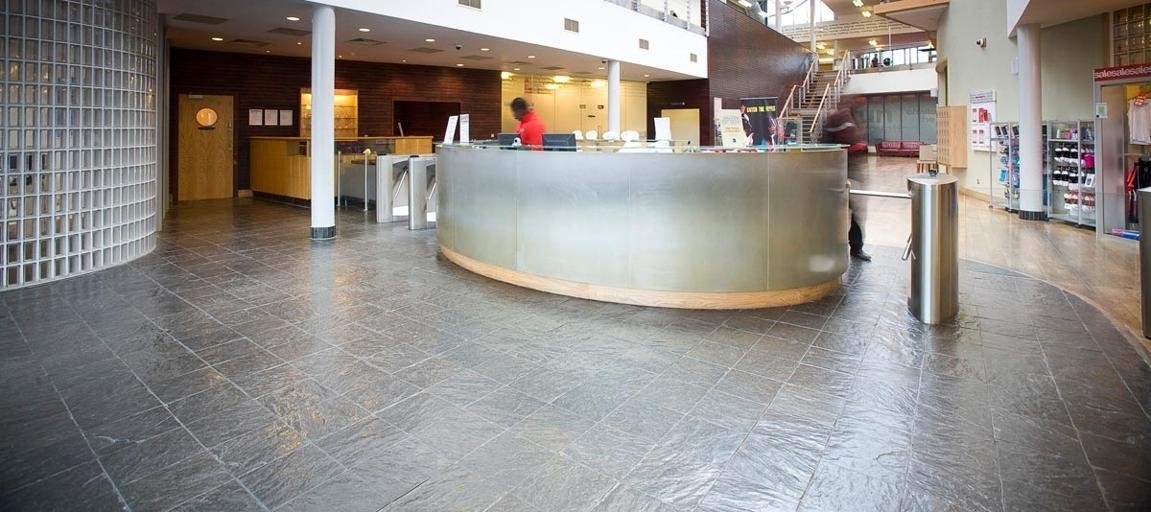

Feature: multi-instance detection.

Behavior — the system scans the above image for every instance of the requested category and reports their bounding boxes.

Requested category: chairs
[917,145,939,173]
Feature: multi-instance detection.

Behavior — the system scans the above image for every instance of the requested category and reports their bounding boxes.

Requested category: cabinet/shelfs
[990,66,1151,240]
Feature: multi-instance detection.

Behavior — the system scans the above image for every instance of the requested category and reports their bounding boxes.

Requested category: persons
[845,178,873,261]
[741,103,757,145]
[510,97,548,150]
[872,53,879,66]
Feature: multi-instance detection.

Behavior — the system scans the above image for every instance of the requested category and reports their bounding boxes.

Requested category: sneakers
[851,247,871,261]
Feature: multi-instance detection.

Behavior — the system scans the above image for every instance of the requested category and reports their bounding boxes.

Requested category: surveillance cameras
[975,37,986,48]
[455,45,462,51]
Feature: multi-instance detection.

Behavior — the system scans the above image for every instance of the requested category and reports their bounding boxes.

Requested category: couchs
[875,139,923,157]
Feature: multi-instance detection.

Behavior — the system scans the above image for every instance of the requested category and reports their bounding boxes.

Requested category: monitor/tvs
[541,133,577,150]
[496,131,520,148]
